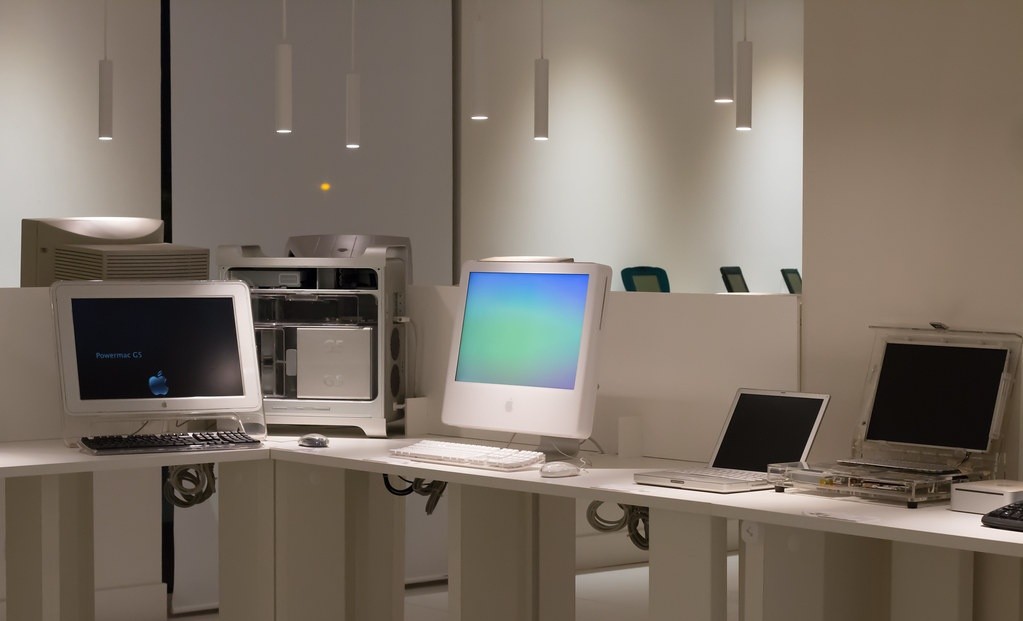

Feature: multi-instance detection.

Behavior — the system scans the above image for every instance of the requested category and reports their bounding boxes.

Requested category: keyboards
[77,430,267,456]
[982,499,1023,532]
[389,440,546,472]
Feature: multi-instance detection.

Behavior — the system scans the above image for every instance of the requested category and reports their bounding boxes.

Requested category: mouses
[541,462,580,477]
[298,433,330,447]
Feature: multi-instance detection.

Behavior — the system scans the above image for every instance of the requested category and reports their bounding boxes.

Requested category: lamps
[533,0,550,140]
[735,0,753,131]
[713,0,735,104]
[275,0,293,134]
[345,0,361,149]
[98,0,113,141]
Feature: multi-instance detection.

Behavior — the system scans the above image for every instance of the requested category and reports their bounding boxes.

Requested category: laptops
[634,388,831,493]
[784,328,1023,492]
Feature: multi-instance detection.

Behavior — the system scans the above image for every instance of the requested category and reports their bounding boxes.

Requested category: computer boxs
[218,244,410,439]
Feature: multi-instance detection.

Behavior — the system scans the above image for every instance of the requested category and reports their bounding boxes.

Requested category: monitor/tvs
[720,266,749,292]
[781,268,802,294]
[20,216,210,288]
[441,259,612,462]
[49,278,268,447]
[620,267,670,293]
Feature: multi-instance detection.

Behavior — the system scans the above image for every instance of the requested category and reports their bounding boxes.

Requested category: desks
[266,429,1023,558]
[0,431,270,479]
